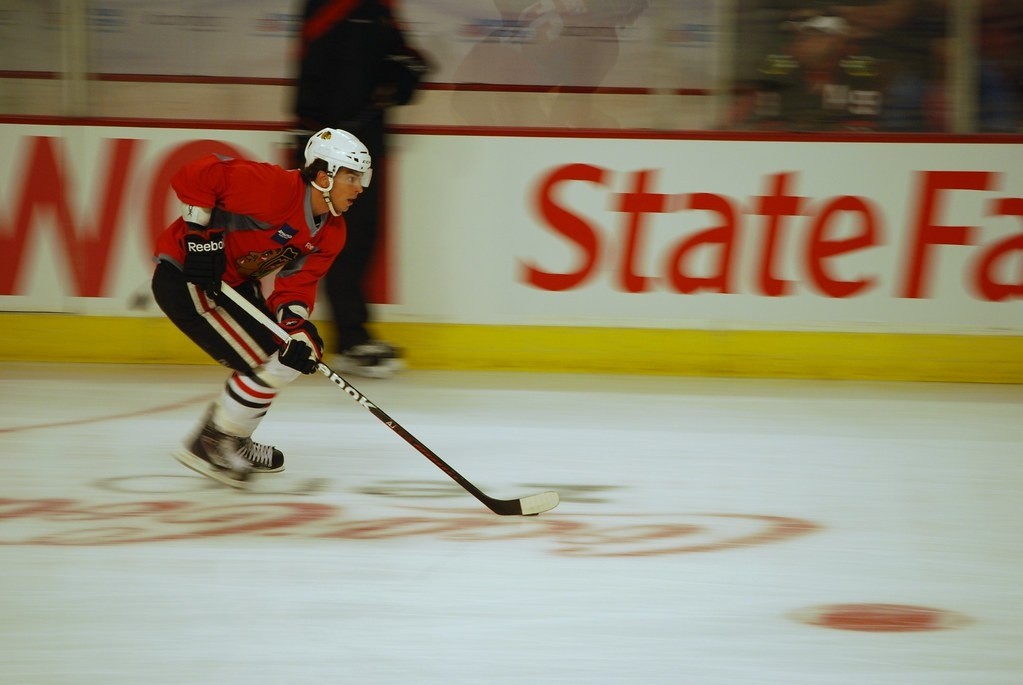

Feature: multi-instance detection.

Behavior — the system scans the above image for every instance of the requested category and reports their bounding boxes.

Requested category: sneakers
[329,332,409,379]
[174,401,253,492]
[237,436,285,474]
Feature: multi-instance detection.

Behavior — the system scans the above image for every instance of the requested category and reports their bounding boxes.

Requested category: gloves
[272,319,323,375]
[177,227,227,299]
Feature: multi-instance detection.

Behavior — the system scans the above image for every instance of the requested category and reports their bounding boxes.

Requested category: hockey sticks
[221,278,560,516]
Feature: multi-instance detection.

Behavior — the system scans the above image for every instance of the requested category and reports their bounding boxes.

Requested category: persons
[151,128,373,487]
[291,1,434,376]
[729,18,882,130]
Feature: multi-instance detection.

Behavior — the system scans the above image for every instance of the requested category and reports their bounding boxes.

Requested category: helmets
[305,127,372,180]
